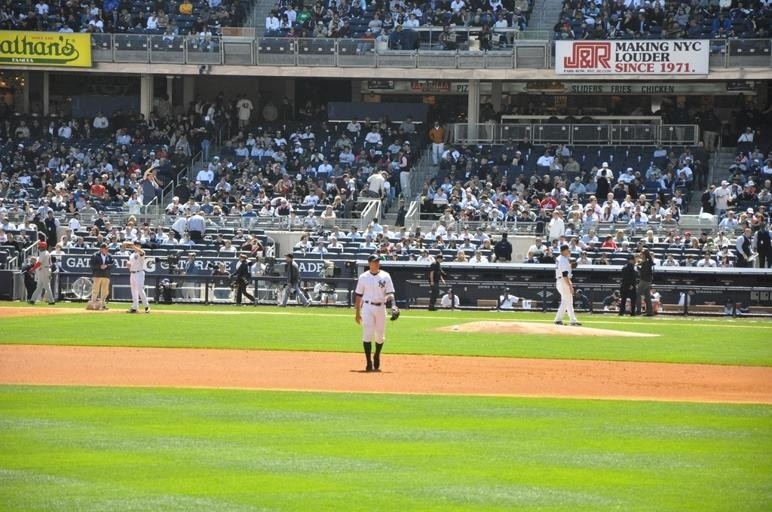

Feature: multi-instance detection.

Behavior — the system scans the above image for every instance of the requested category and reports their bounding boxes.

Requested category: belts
[365,301,381,305]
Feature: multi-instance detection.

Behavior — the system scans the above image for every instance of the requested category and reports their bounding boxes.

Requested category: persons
[354,252,400,373]
[552,243,583,327]
[1,0,772,315]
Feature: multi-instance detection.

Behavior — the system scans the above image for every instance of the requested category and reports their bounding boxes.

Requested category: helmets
[37,242,48,250]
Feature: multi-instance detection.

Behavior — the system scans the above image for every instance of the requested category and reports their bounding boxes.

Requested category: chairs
[52,225,268,256]
[437,145,699,202]
[293,231,495,261]
[534,235,739,267]
[0,228,37,270]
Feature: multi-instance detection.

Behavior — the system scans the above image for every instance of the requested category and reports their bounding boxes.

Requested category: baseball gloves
[390,305,400,320]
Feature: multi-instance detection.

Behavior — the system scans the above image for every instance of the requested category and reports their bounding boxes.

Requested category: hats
[368,254,381,262]
[134,240,142,246]
[100,243,110,249]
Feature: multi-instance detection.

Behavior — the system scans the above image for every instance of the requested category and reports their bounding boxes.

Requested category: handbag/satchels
[242,273,252,285]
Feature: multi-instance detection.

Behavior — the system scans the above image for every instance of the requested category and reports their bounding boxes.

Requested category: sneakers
[429,308,437,311]
[144,306,150,313]
[127,308,136,313]
[254,298,258,307]
[102,306,109,310]
[554,321,567,325]
[366,361,372,370]
[278,304,286,308]
[569,322,581,326]
[234,303,242,306]
[48,302,55,305]
[301,302,309,309]
[373,353,379,369]
[28,300,34,305]
